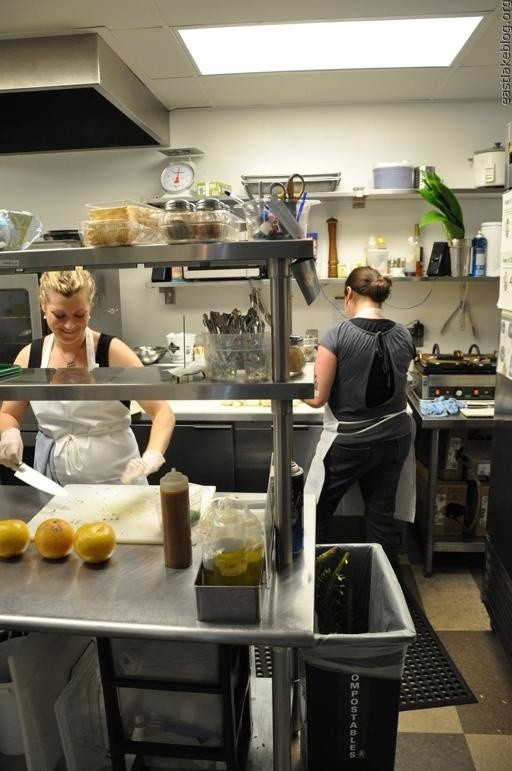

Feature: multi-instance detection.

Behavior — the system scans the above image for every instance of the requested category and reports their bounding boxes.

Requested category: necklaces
[53,340,86,369]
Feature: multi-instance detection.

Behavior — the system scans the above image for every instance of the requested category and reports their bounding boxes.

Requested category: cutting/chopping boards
[24,483,216,546]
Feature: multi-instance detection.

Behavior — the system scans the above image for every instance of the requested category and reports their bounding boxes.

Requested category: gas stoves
[408,343,499,401]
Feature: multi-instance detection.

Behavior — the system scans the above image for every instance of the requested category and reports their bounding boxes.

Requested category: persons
[303,268,416,592]
[1,268,176,484]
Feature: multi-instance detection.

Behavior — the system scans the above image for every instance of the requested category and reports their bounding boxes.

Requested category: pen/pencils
[260,180,266,225]
[241,182,259,215]
[224,190,255,217]
[296,187,309,223]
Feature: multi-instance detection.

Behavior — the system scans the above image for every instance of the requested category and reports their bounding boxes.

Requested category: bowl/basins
[192,499,274,626]
[0,209,33,252]
[201,331,274,384]
[242,173,343,180]
[131,346,169,365]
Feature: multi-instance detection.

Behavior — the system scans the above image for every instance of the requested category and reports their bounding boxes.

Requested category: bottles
[470,232,487,276]
[291,461,303,554]
[408,223,423,276]
[193,197,220,239]
[165,199,193,239]
[160,470,192,568]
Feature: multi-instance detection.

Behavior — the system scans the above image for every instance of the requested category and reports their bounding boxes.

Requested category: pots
[468,142,510,188]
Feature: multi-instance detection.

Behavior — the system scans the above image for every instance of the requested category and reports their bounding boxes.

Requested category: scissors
[269,173,305,218]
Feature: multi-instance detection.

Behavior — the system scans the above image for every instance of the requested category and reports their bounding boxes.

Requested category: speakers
[427,242,451,277]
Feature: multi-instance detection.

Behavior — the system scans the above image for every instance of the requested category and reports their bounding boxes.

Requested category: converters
[414,324,424,337]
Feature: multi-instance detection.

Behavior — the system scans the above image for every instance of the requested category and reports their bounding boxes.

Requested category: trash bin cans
[297,543,417,770]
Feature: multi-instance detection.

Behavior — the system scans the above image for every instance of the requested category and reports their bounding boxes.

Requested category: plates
[243,180,340,195]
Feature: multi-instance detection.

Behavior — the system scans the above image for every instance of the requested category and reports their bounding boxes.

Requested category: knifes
[1,456,72,498]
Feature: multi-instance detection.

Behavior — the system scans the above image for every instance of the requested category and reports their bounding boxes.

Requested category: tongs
[455,279,473,332]
[438,300,481,337]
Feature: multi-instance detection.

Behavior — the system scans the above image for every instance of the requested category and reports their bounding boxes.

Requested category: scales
[159,147,204,196]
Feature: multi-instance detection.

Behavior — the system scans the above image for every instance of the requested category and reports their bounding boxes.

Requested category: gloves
[117,447,167,485]
[0,427,25,469]
